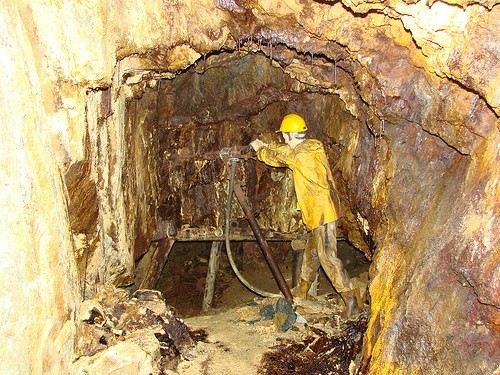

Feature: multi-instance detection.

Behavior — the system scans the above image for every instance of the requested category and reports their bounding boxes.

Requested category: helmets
[275,113,308,132]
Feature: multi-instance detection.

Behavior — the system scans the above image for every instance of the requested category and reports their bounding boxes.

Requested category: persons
[249,114,366,322]
